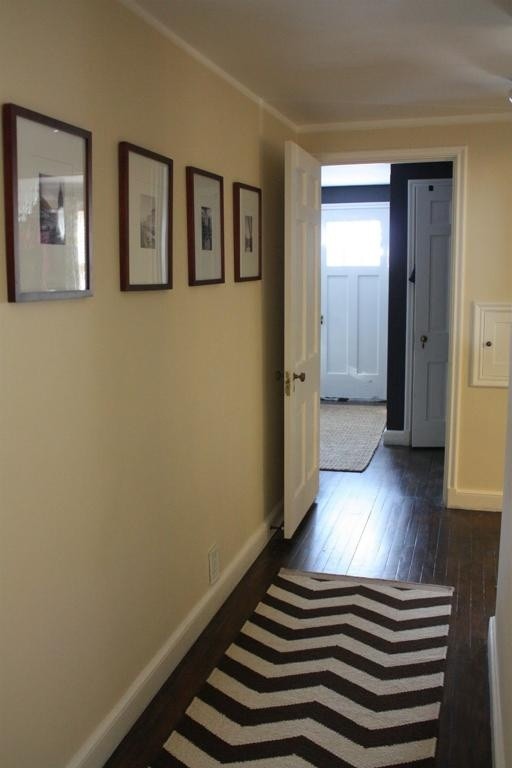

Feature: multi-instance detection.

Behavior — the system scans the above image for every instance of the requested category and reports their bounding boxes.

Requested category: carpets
[143,566,454,768]
[319,400,386,472]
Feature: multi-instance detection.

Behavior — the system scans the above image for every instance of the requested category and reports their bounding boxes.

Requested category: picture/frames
[4,102,92,303]
[185,166,226,287]
[233,181,262,282]
[119,140,173,290]
